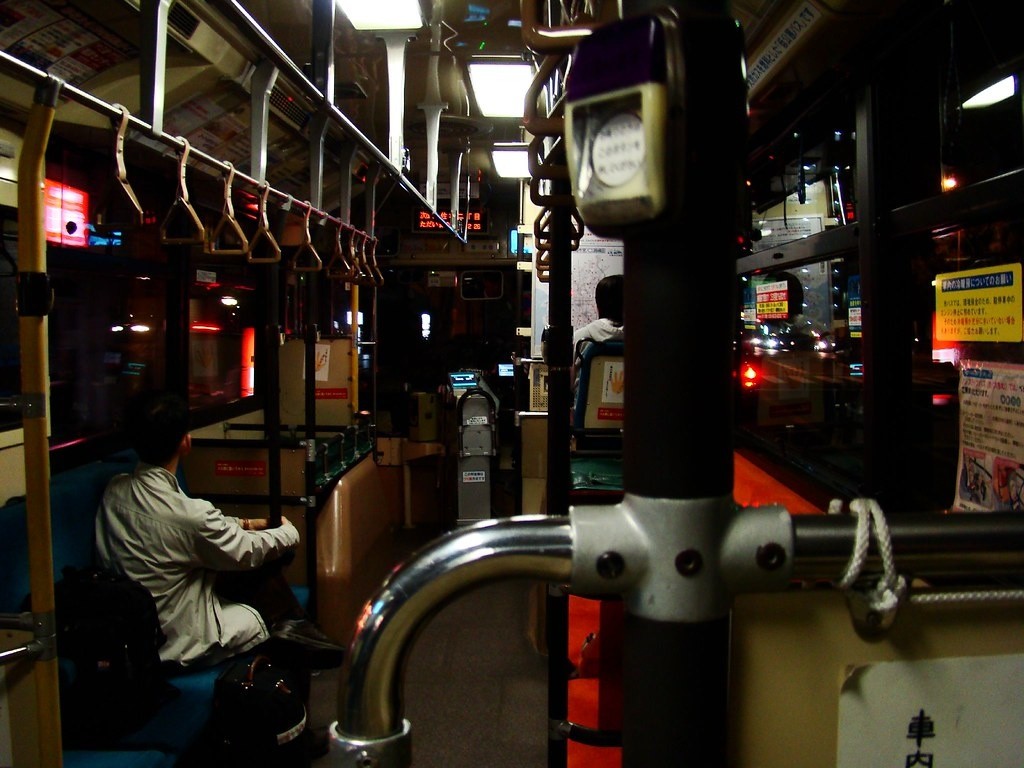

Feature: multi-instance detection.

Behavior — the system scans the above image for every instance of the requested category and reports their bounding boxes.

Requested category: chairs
[572,336,626,447]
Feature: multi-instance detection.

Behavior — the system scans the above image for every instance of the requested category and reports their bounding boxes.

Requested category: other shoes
[270,616,347,653]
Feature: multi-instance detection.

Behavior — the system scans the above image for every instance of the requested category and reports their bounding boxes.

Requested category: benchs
[0,447,309,768]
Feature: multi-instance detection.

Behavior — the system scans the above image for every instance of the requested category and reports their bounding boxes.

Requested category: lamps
[490,142,539,178]
[335,0,429,31]
[461,18,536,124]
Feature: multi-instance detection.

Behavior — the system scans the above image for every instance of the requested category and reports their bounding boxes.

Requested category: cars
[842,348,863,386]
[723,312,834,390]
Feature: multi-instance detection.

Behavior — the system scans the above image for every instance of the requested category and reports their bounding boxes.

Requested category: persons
[762,271,830,353]
[94,402,348,768]
[570,273,626,439]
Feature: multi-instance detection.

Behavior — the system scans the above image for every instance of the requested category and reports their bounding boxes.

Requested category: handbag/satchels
[206,652,313,768]
